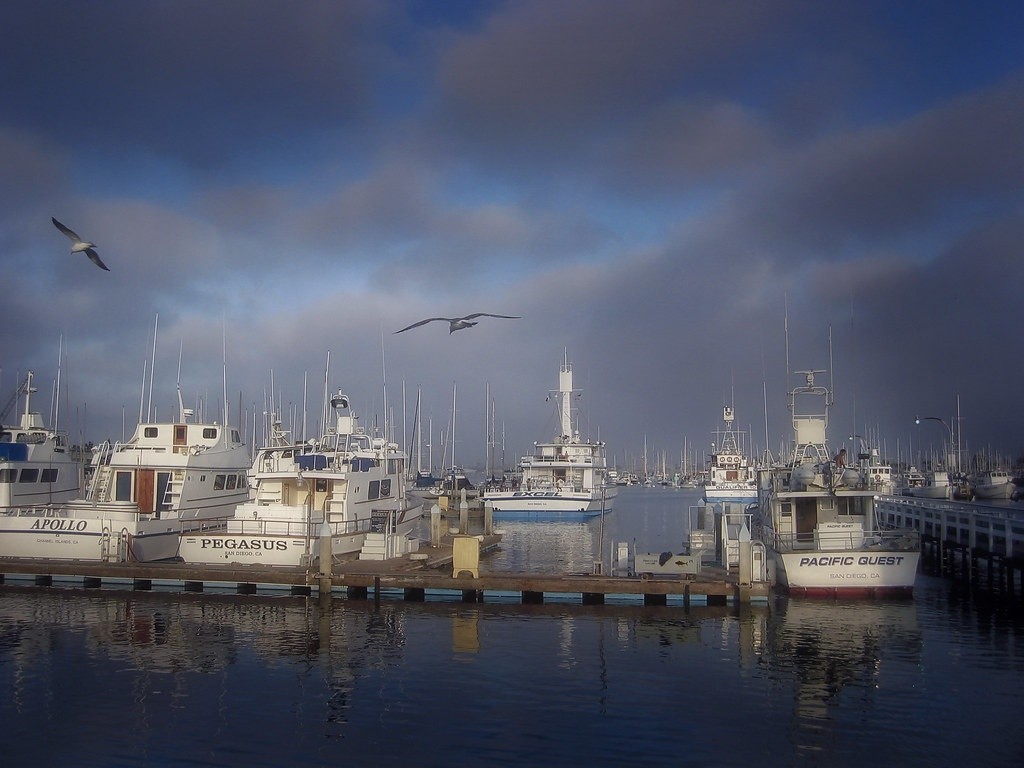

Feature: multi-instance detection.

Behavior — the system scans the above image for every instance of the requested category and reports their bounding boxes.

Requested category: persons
[834,449,846,468]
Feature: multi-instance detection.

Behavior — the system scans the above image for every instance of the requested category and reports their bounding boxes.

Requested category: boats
[173,348,426,568]
[743,380,924,603]
[0,310,253,564]
[475,346,619,523]
[604,293,1024,507]
[247,326,526,518]
[0,333,87,517]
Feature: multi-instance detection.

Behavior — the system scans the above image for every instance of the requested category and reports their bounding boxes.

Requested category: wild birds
[51,216,111,272]
[392,312,523,336]
